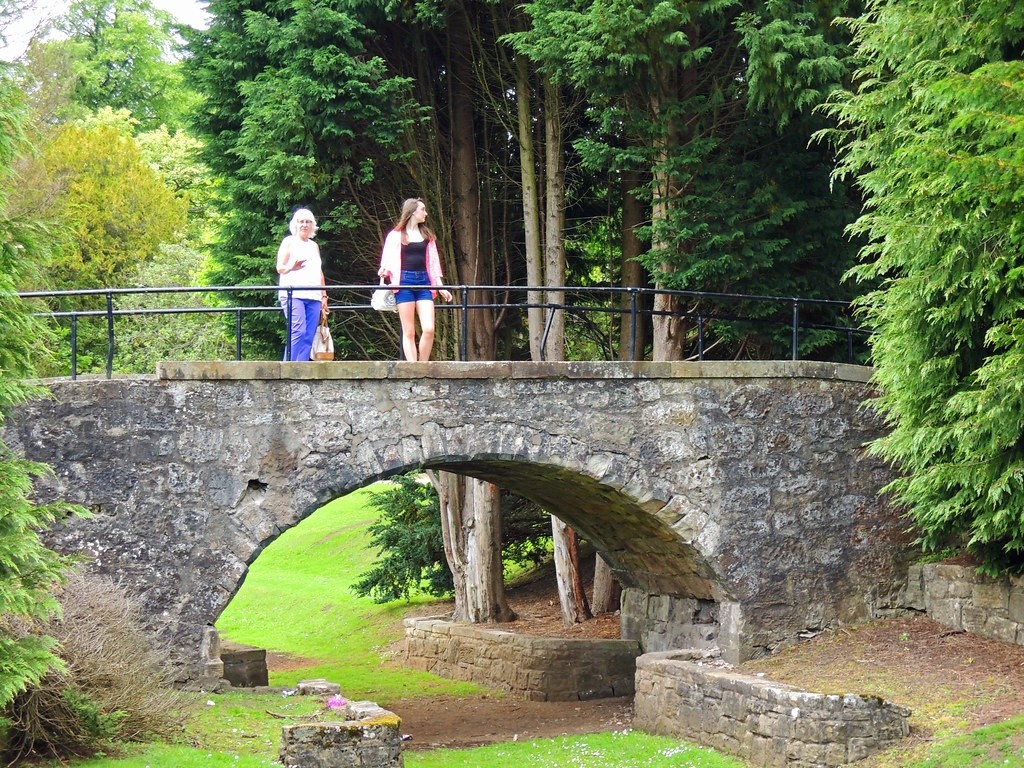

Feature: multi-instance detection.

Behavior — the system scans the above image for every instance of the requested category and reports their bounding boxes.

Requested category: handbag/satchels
[310,310,334,361]
[370,276,399,312]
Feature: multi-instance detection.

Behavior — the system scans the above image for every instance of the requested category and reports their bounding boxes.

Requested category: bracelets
[323,295,329,299]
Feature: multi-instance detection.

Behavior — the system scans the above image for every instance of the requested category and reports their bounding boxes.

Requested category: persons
[273,208,330,364]
[375,197,454,364]
